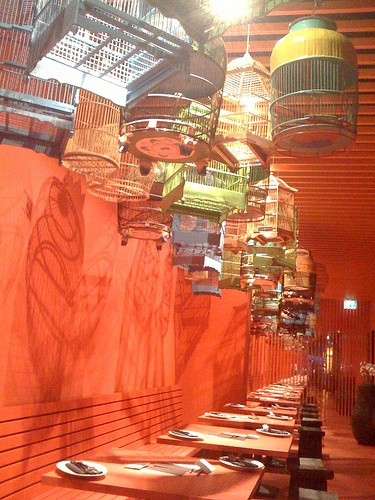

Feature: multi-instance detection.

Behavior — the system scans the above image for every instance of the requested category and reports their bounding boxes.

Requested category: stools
[289,404,338,500]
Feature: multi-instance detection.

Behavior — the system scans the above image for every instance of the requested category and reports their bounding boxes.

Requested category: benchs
[0,384,201,500]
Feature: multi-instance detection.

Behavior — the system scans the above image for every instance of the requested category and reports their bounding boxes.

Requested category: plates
[267,414,293,420]
[219,456,263,469]
[231,405,245,407]
[56,460,108,476]
[169,430,205,439]
[207,412,230,418]
[256,428,290,436]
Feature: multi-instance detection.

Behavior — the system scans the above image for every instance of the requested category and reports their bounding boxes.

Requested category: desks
[41,382,304,500]
